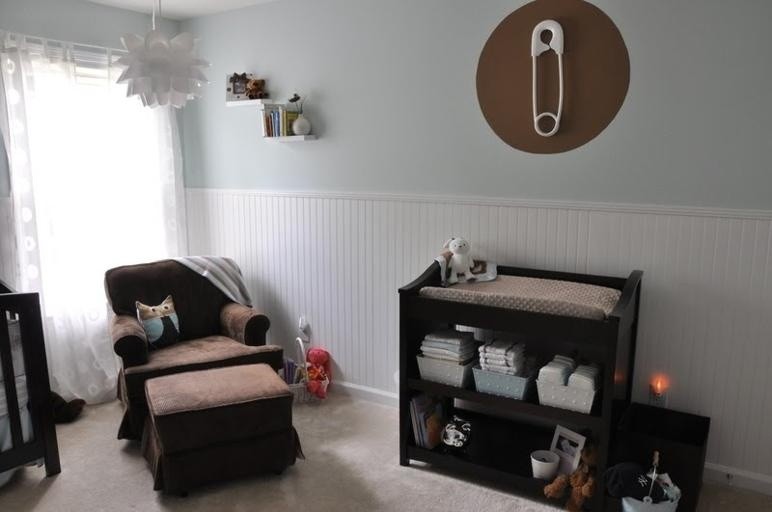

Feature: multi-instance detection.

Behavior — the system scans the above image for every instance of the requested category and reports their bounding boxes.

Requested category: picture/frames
[550,424,587,477]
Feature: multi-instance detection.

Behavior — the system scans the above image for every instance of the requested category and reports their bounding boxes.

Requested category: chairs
[104,255,286,442]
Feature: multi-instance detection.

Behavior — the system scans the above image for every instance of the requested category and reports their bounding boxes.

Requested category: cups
[529,451,560,482]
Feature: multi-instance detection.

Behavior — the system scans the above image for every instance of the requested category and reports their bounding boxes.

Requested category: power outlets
[648,384,668,408]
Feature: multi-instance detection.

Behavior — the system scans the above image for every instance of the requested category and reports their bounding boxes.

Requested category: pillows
[134,294,184,352]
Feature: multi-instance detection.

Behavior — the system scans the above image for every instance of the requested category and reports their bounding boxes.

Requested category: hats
[607,462,664,502]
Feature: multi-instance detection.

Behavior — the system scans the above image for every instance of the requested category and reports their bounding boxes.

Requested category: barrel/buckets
[623,449,681,511]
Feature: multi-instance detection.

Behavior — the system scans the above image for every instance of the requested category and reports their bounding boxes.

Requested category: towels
[477,337,541,376]
[538,354,600,391]
[419,330,477,365]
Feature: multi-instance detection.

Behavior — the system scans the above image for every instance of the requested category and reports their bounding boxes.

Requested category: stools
[140,363,306,498]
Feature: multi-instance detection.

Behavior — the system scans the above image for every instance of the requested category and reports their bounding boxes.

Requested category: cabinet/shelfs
[223,73,316,142]
[398,251,644,512]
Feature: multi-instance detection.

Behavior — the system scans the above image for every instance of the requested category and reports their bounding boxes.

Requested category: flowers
[288,93,309,113]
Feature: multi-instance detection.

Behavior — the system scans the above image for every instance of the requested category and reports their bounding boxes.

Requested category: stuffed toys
[245,79,266,99]
[542,438,603,512]
[300,348,333,399]
[443,236,477,286]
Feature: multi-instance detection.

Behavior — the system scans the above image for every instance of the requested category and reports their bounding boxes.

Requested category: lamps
[108,1,212,110]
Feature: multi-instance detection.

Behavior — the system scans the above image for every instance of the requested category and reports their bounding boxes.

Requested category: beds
[0,279,62,477]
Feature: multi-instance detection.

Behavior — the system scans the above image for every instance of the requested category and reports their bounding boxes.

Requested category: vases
[292,114,312,135]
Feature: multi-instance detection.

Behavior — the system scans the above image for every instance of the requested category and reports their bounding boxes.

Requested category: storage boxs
[471,365,540,401]
[536,379,602,415]
[416,353,479,389]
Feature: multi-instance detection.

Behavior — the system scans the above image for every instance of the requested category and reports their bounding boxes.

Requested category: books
[264,104,299,137]
[409,395,445,449]
